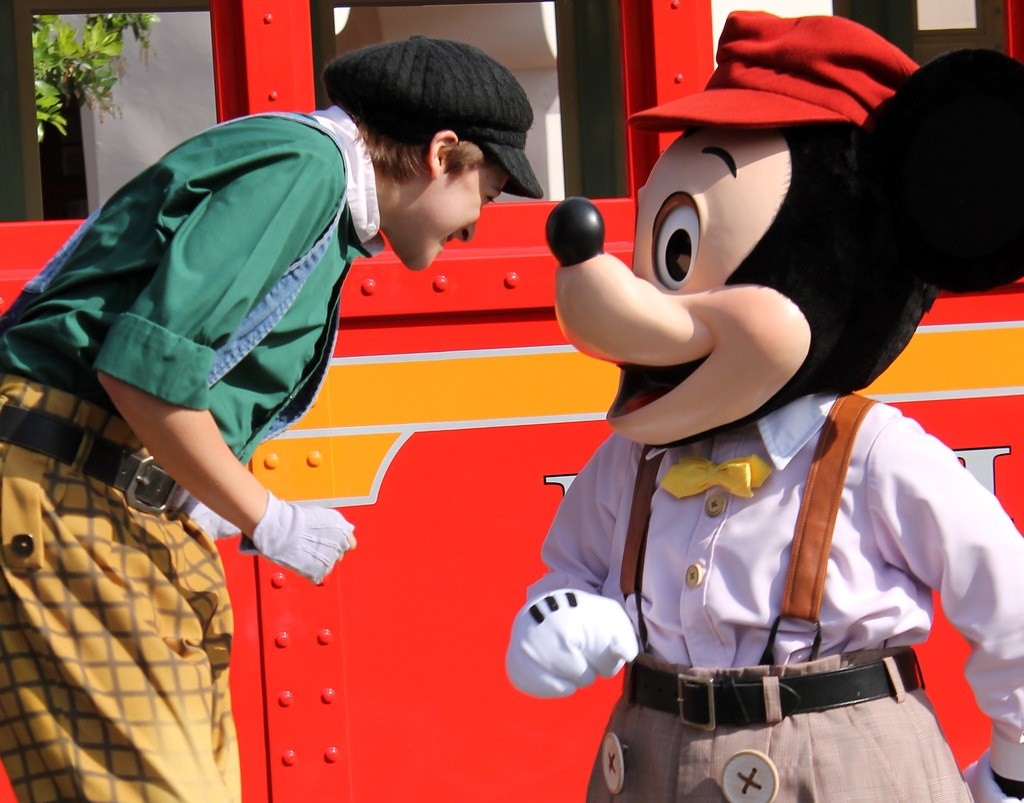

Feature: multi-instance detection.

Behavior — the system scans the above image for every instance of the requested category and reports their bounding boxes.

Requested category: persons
[0,34,543,803]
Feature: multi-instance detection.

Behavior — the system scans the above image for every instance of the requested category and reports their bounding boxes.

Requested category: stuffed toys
[505,11,1024,803]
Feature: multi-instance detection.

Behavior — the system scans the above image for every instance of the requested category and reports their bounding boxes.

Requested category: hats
[323,35,544,200]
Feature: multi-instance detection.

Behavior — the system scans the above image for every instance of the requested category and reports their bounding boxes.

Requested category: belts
[0,406,201,520]
